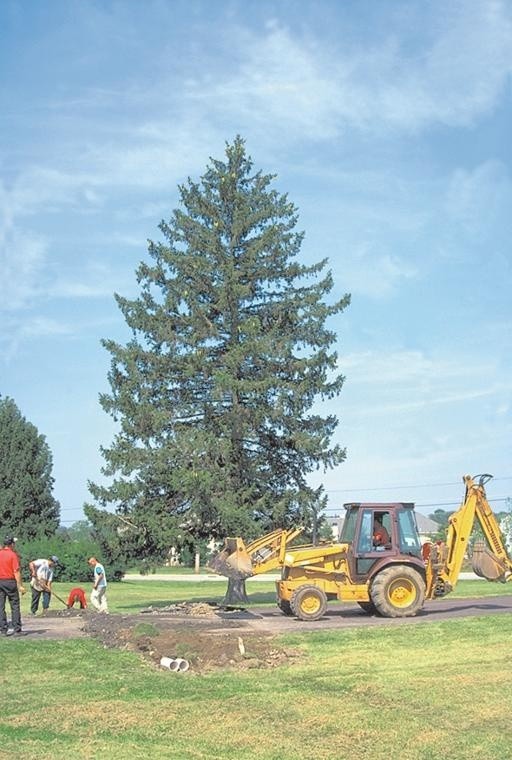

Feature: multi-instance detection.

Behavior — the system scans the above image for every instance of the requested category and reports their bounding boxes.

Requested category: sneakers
[11,630,28,638]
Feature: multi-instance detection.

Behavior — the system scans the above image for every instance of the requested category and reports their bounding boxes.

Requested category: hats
[2,535,19,546]
[49,556,60,567]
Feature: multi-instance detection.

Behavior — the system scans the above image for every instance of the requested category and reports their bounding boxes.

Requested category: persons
[88,556,110,616]
[68,587,88,608]
[28,555,61,618]
[0,535,30,639]
[372,516,392,550]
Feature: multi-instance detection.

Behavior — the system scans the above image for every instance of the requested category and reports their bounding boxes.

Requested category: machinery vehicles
[203,473,512,622]
